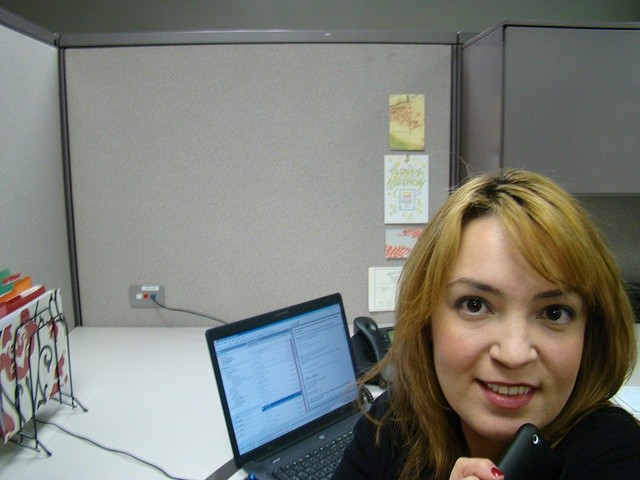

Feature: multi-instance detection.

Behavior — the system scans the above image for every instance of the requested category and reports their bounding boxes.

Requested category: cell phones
[496,422,558,480]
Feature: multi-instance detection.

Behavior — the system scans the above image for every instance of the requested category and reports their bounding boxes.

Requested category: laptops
[205,292,373,480]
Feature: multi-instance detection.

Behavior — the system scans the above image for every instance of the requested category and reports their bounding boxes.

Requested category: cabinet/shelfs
[458,21,639,195]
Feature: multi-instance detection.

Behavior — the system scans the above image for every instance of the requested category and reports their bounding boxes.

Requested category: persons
[328,167,640,480]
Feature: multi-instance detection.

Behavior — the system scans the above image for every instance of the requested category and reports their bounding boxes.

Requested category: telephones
[347,317,396,402]
